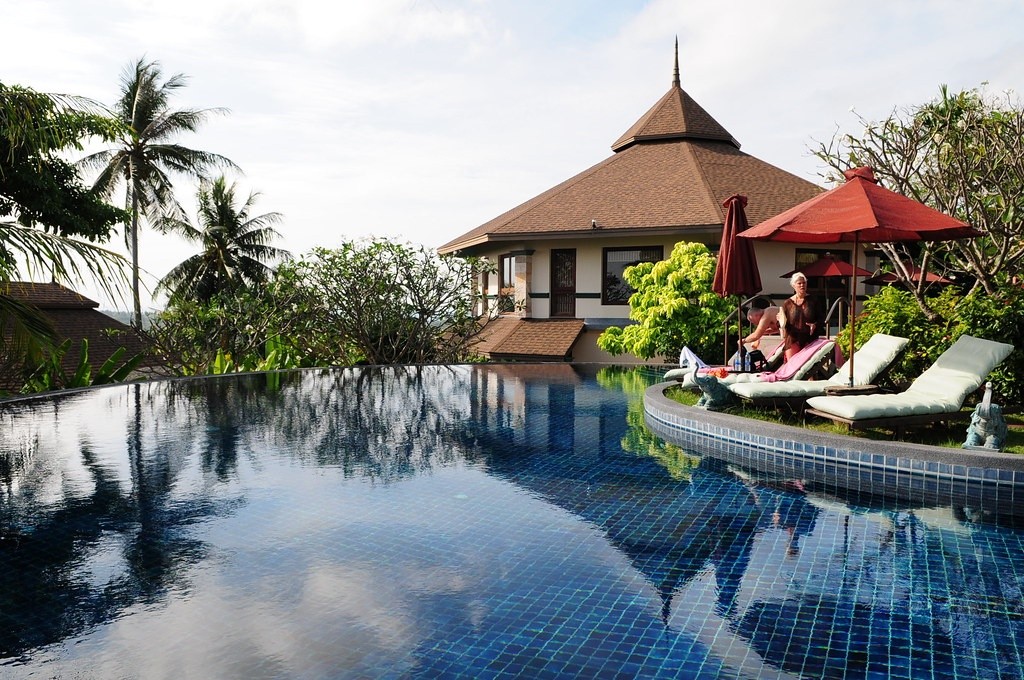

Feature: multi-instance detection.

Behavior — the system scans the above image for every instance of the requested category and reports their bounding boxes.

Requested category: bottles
[745,352,750,371]
[735,351,741,371]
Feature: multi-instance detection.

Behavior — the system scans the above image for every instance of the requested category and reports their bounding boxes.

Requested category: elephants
[961,382,1008,450]
[693,361,739,411]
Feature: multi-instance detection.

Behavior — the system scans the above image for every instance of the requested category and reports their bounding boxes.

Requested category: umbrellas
[778,254,876,340]
[711,193,763,356]
[860,259,958,289]
[734,165,990,388]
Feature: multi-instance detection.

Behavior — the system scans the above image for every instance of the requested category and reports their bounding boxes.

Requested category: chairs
[804,334,1014,433]
[681,338,836,394]
[663,336,785,382]
[726,332,912,405]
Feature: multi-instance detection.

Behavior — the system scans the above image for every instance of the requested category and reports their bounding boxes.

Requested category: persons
[737,306,781,343]
[776,272,825,362]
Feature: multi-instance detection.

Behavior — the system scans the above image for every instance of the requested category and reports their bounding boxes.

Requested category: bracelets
[744,338,746,343]
